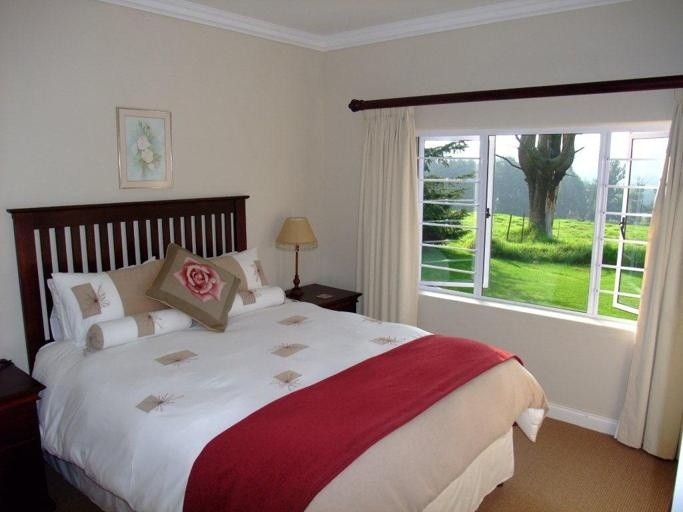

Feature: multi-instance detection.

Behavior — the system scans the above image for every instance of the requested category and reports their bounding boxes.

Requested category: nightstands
[285,282,363,314]
[0,359,56,512]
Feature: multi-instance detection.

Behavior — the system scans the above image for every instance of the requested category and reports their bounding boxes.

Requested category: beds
[6,193,550,511]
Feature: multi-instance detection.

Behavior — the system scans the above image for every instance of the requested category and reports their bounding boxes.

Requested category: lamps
[274,217,318,300]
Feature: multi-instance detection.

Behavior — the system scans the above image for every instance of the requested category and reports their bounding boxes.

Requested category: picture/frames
[117,106,173,191]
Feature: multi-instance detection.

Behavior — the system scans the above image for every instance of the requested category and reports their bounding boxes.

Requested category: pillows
[44,243,286,360]
